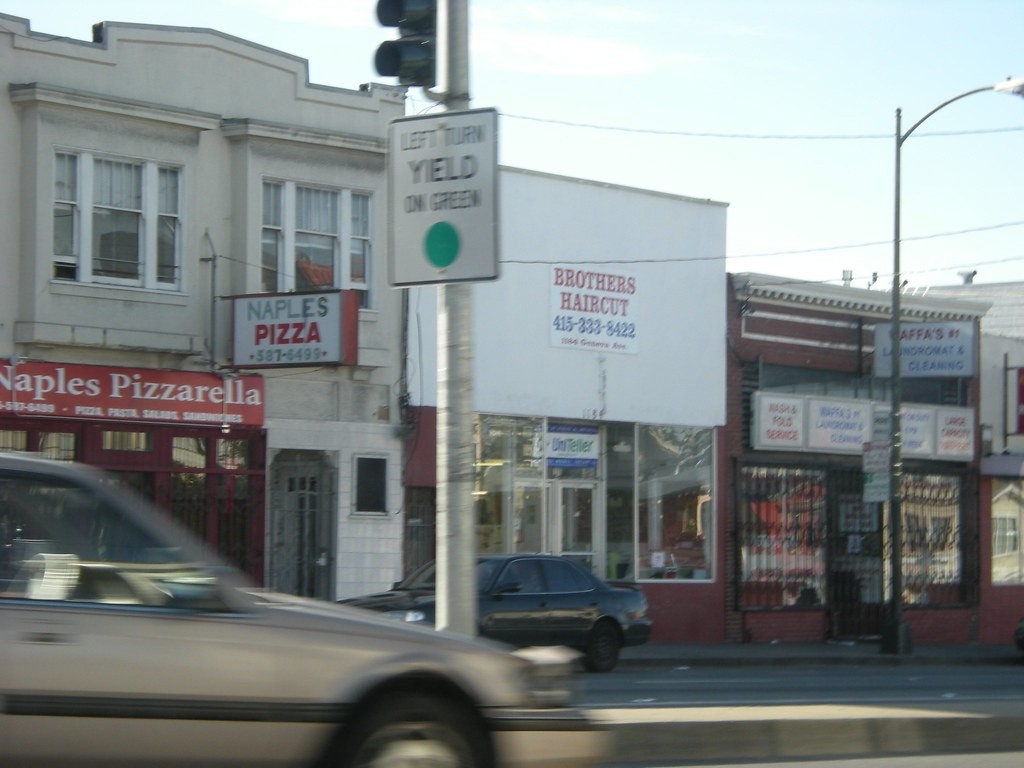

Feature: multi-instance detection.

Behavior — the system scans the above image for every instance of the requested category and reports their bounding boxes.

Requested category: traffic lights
[374,0,437,87]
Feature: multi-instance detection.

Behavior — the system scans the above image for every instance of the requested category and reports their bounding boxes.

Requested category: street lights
[885,75,1024,657]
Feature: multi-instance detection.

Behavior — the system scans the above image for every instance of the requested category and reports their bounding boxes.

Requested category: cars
[334,551,654,673]
[0,449,621,768]
